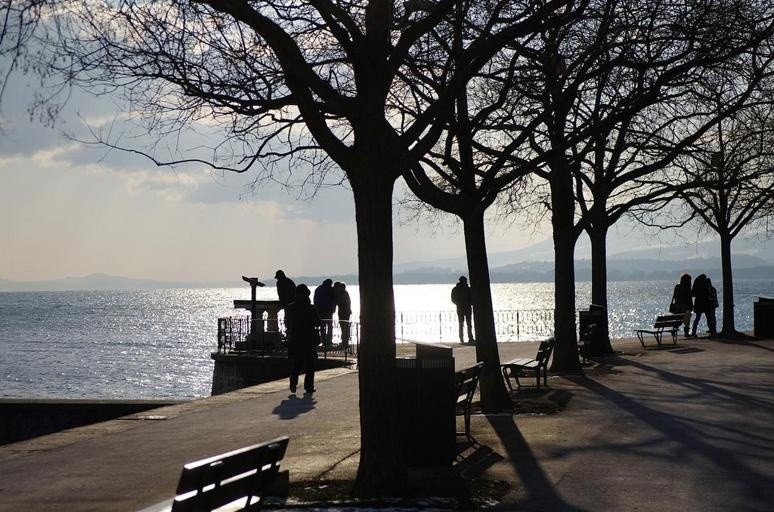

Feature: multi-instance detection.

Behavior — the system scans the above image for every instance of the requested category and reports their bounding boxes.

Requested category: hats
[458,276,467,283]
[292,284,312,301]
[274,270,284,277]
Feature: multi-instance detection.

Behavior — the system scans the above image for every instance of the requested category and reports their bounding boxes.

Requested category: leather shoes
[692,330,698,337]
[683,326,691,337]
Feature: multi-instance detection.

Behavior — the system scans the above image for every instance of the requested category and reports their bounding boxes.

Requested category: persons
[450,276,476,345]
[284,283,321,393]
[334,281,352,350]
[274,269,296,310]
[313,278,336,347]
[673,273,719,337]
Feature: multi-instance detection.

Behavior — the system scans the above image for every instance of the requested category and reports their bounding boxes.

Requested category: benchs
[500,337,557,392]
[150,433,289,512]
[450,361,488,453]
[633,313,689,349]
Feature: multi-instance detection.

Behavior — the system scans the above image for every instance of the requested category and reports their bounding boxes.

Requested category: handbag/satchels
[669,294,678,314]
[297,335,320,354]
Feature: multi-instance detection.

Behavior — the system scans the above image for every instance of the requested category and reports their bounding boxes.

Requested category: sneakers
[306,388,316,393]
[321,342,350,350]
[290,377,296,393]
